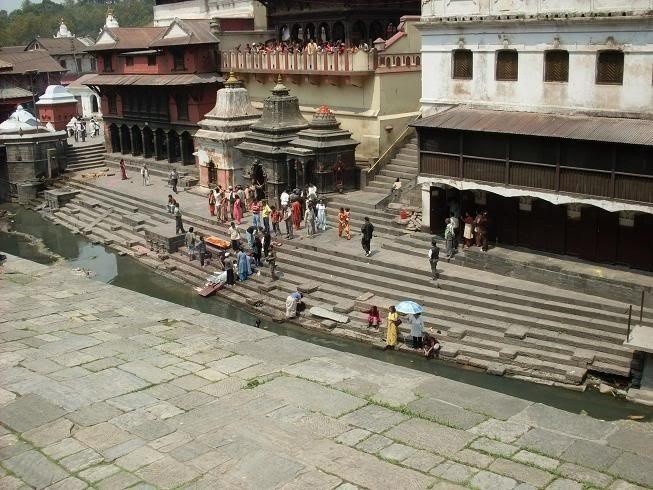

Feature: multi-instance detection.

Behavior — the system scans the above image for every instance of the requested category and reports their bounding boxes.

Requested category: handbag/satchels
[393,318,403,327]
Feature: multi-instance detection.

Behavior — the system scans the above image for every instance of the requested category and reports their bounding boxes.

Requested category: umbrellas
[395,300,423,319]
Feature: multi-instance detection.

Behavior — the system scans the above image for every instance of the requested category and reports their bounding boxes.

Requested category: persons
[443,207,490,261]
[72,113,101,142]
[427,240,440,280]
[360,306,382,329]
[166,194,176,214]
[407,313,425,351]
[423,332,440,358]
[360,217,375,257]
[234,38,372,57]
[386,305,399,347]
[186,180,352,287]
[286,291,303,318]
[141,163,149,187]
[390,177,402,193]
[119,159,128,179]
[174,203,186,235]
[169,168,179,195]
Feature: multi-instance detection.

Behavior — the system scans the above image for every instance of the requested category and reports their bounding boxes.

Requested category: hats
[224,252,231,257]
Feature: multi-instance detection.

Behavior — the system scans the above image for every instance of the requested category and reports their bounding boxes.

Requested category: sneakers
[366,251,371,257]
